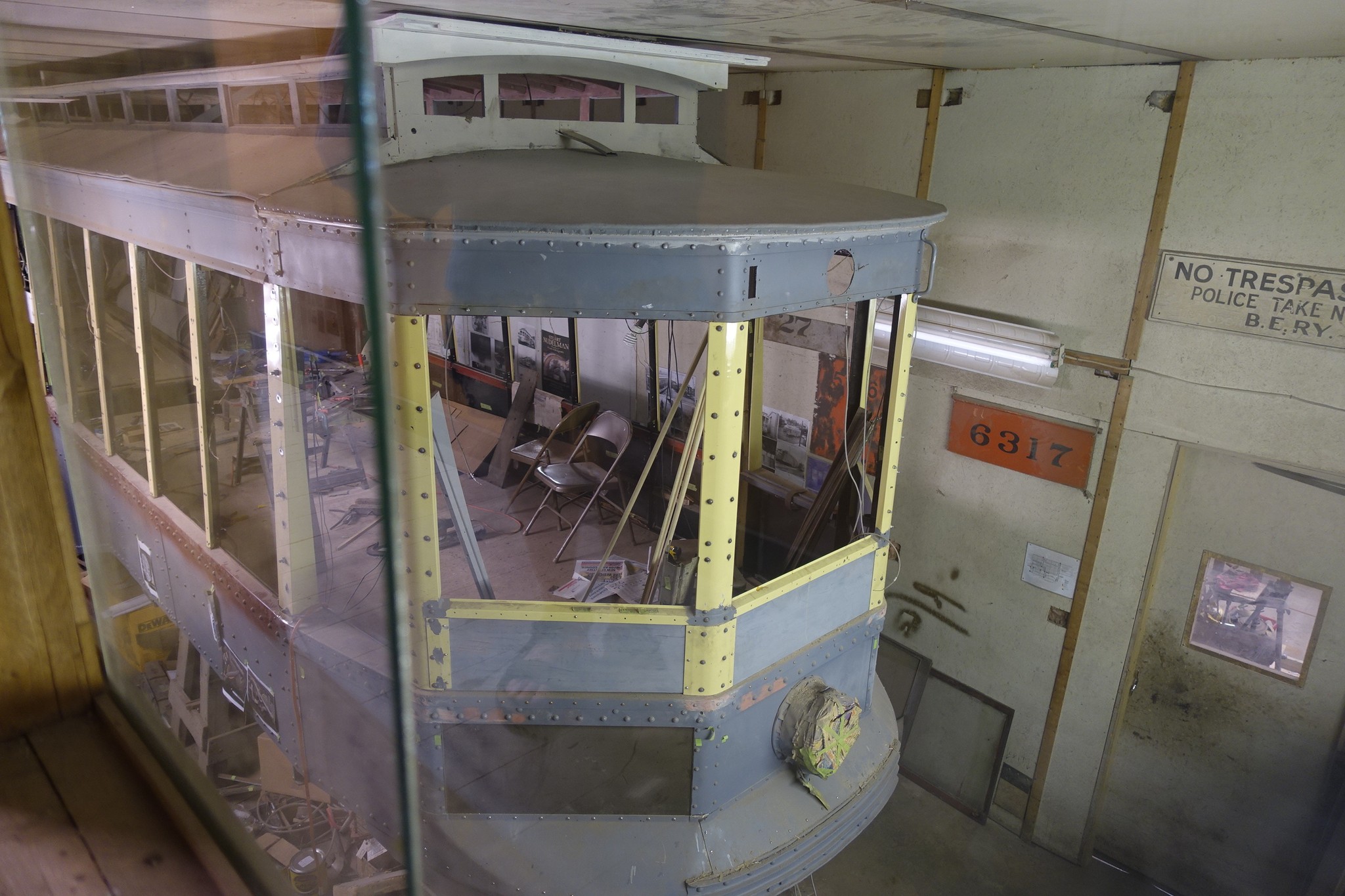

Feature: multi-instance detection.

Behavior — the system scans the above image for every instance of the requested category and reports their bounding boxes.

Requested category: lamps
[870,292,1068,396]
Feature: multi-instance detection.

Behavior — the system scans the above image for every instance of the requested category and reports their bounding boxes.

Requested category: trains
[0,0,950,895]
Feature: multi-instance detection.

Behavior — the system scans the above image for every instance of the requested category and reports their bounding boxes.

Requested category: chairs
[519,409,636,564]
[503,398,603,532]
